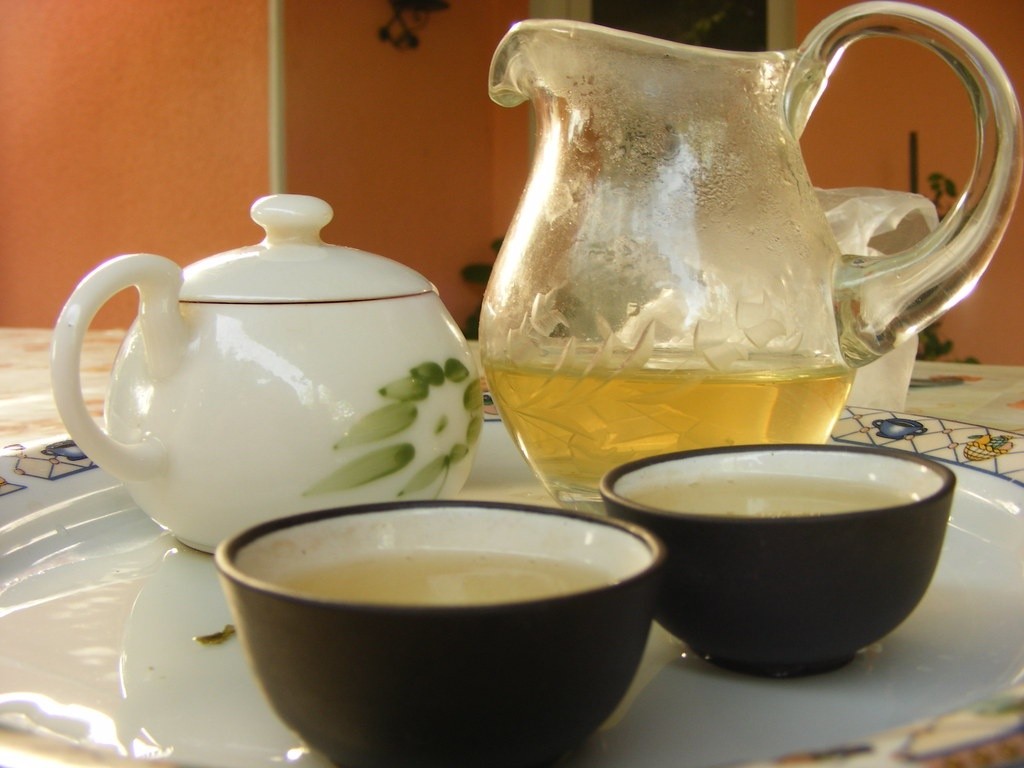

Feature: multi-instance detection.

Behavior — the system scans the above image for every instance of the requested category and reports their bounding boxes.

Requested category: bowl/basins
[601,444,956,678]
[214,501,665,768]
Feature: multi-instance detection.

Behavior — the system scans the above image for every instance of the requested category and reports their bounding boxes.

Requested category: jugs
[478,0,1024,514]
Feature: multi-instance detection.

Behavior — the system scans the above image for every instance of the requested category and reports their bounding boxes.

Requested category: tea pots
[52,195,485,555]
